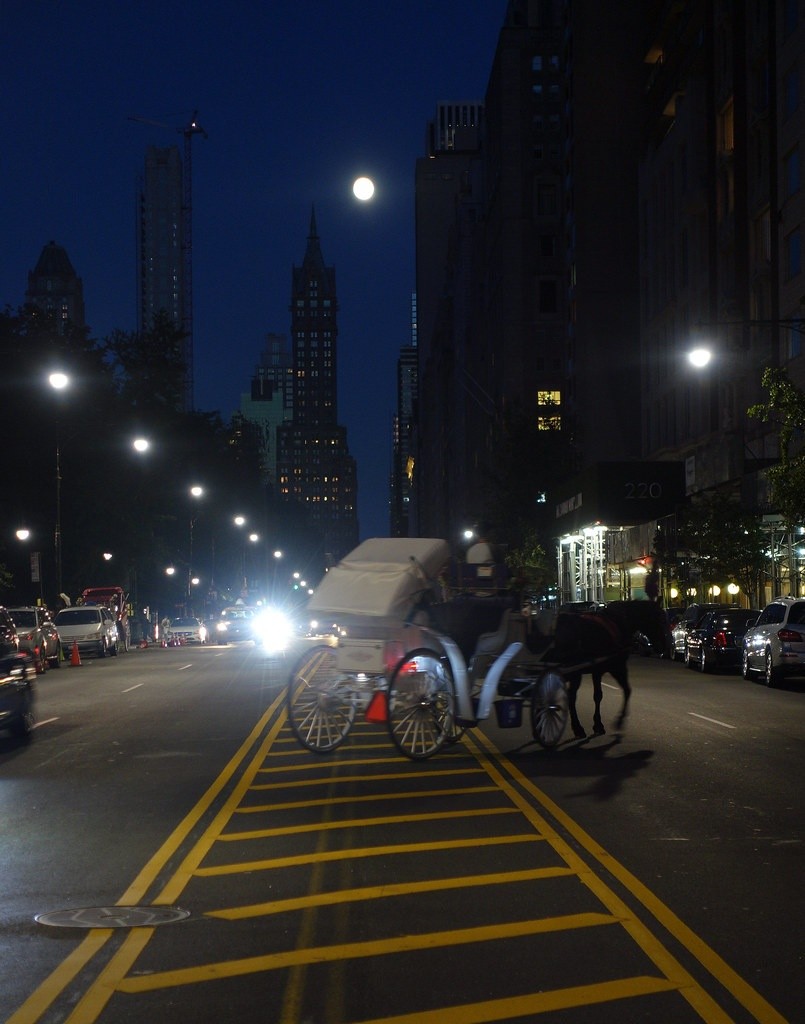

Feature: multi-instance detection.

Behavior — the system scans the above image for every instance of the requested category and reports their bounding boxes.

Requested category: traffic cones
[69,640,84,668]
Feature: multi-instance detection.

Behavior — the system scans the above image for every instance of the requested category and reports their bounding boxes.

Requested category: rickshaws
[80,587,130,653]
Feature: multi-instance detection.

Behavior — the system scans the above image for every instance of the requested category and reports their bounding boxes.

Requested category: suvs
[742,594,805,690]
[669,602,741,661]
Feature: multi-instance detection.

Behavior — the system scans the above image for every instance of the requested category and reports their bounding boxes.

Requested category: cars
[684,608,761,672]
[638,607,683,655]
[0,604,37,746]
[5,605,60,672]
[168,617,209,645]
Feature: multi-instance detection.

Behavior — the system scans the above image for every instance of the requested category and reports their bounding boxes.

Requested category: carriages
[283,538,675,761]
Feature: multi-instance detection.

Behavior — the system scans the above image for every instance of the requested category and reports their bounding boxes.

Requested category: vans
[52,606,118,659]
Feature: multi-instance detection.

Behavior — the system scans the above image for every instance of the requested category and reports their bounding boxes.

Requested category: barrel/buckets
[455,698,480,728]
[494,695,525,728]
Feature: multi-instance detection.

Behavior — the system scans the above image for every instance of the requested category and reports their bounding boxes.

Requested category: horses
[529,599,676,738]
